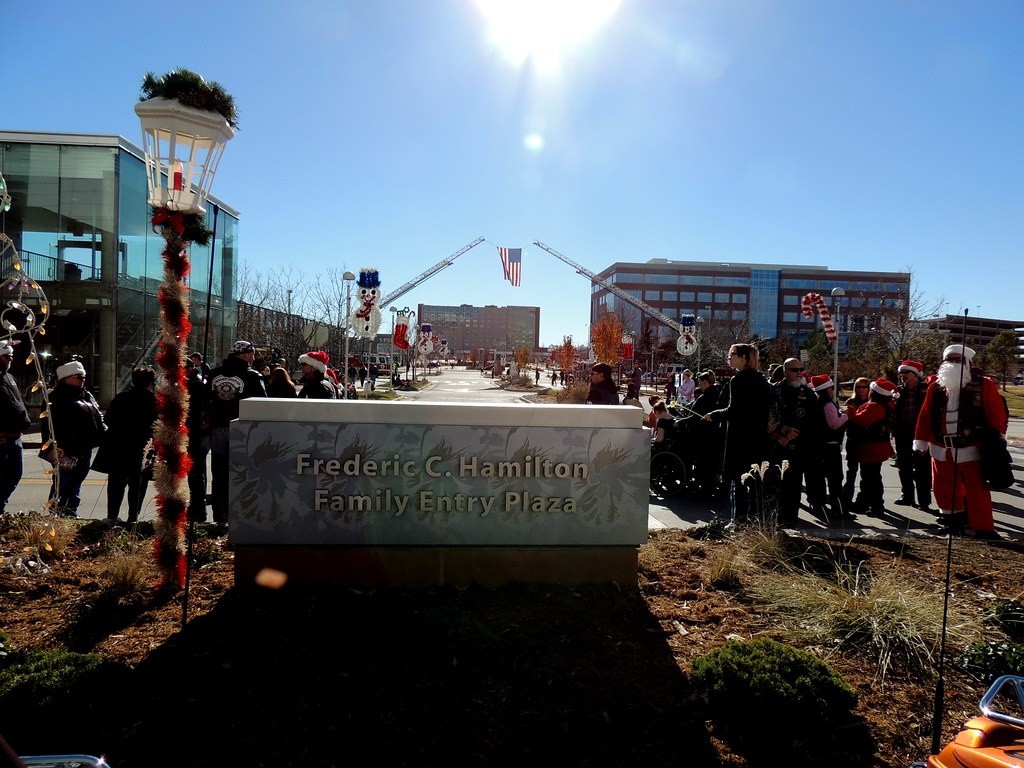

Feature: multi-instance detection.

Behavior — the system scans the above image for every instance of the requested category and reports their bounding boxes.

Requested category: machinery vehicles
[340,235,485,331]
[532,238,699,332]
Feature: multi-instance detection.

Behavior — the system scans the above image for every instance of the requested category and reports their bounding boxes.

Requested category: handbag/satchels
[38,439,78,471]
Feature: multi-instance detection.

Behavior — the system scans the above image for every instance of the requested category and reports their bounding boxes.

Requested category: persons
[359,365,367,387]
[560,371,565,385]
[623,359,645,410]
[911,345,1008,541]
[186,338,358,529]
[551,370,557,386]
[535,369,540,384]
[89,367,159,523]
[392,374,401,386]
[369,364,378,383]
[40,361,106,520]
[0,339,31,515]
[564,373,568,385]
[585,362,619,405]
[841,360,933,514]
[348,364,358,384]
[648,342,858,528]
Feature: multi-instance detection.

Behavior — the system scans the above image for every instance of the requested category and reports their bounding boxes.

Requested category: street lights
[696,316,704,372]
[342,271,356,400]
[384,306,397,393]
[830,287,846,403]
[134,68,236,590]
[413,325,420,384]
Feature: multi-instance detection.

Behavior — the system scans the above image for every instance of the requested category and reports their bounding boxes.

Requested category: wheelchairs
[648,426,686,499]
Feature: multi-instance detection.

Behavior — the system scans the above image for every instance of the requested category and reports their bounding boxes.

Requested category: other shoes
[894,496,914,505]
[189,514,208,523]
[866,507,884,517]
[831,510,855,520]
[919,504,928,510]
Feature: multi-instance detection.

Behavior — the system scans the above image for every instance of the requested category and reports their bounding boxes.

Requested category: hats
[56,361,86,379]
[869,379,901,399]
[229,341,255,355]
[810,376,834,392]
[299,350,330,374]
[0,339,14,357]
[941,345,976,362]
[324,367,343,391]
[898,360,924,377]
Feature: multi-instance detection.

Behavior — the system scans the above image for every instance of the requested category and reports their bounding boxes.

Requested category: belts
[934,432,970,449]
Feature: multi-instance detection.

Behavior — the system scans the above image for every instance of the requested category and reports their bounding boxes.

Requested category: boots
[973,528,1002,540]
[934,512,965,537]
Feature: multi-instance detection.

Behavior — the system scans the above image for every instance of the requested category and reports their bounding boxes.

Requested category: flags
[496,246,521,287]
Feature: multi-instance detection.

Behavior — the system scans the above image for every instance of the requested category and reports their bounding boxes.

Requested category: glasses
[591,371,601,376]
[4,354,14,358]
[856,385,868,390]
[70,375,86,380]
[900,370,911,375]
[784,368,805,373]
